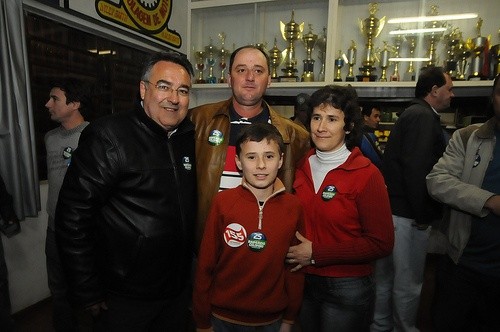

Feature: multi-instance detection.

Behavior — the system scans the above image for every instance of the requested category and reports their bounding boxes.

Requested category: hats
[294,93,311,111]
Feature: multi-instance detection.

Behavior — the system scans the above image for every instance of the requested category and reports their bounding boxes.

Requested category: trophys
[334,1,500,82]
[195,10,326,84]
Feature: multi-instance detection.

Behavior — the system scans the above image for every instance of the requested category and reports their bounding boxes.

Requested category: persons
[195,121,306,332]
[43,76,90,332]
[54,50,197,332]
[187,46,312,255]
[384,66,456,332]
[286,84,395,332]
[0,173,22,332]
[426,72,500,332]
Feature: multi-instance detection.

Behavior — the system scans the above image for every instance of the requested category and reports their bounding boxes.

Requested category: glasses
[144,79,192,96]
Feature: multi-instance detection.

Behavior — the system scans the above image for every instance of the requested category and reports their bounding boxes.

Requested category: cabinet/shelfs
[186,0,500,88]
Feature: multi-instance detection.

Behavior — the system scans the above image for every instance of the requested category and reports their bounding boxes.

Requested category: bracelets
[310,254,316,265]
